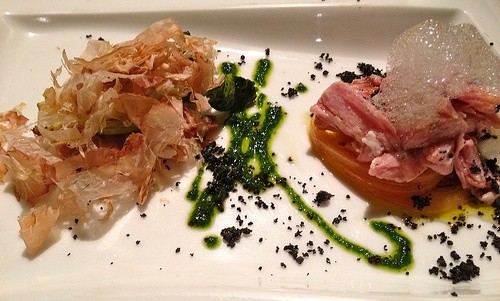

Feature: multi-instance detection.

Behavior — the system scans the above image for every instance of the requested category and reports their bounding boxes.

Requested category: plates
[0,0,499,300]
